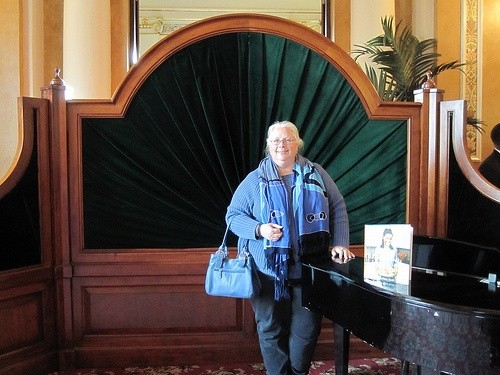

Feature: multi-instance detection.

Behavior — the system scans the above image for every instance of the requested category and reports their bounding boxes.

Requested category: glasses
[269,137,297,145]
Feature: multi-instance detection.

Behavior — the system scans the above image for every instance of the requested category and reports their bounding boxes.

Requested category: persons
[477,123,500,187]
[376,229,399,281]
[225,121,355,375]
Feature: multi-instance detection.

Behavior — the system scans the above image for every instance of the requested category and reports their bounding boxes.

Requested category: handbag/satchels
[205,214,261,298]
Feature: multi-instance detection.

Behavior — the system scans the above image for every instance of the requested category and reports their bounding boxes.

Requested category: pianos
[298,233,499,375]
